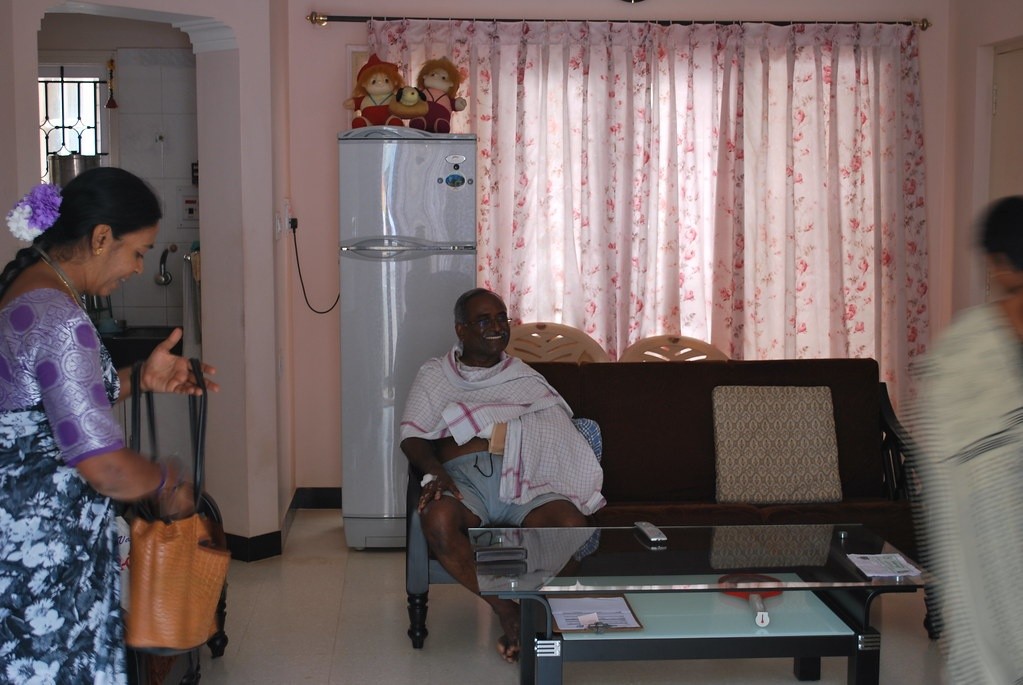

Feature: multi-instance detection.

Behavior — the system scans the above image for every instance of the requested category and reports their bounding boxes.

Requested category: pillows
[712,386,843,504]
[709,525,833,567]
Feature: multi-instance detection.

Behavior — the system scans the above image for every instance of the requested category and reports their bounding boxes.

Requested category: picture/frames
[347,43,370,127]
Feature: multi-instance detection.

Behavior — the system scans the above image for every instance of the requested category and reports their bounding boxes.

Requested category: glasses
[464,314,512,328]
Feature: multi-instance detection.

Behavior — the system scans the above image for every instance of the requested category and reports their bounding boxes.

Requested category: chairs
[504,323,730,361]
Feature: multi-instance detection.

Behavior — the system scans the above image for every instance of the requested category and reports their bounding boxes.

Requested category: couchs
[406,357,944,650]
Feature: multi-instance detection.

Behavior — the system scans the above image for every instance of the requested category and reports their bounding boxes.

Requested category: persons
[914,194,1023,685]
[399,287,607,664]
[0,166,218,685]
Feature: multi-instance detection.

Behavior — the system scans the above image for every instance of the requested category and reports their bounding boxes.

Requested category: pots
[48,151,99,191]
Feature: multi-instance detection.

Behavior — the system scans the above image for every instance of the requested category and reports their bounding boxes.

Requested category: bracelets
[156,463,168,498]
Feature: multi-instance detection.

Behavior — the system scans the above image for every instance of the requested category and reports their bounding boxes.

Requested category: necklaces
[41,256,78,306]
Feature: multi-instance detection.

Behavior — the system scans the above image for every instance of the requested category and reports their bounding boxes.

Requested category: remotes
[634,521,667,545]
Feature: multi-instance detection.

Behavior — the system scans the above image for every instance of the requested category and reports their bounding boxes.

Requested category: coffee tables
[468,528,939,685]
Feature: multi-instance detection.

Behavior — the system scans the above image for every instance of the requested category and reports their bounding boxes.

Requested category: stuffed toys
[343,53,467,134]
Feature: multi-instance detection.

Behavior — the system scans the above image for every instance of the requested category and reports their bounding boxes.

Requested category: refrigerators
[336,125,477,551]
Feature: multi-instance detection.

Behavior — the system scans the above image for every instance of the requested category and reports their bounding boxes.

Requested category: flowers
[5,184,62,241]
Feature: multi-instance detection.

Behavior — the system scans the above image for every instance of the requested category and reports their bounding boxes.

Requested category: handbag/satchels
[125,357,231,650]
